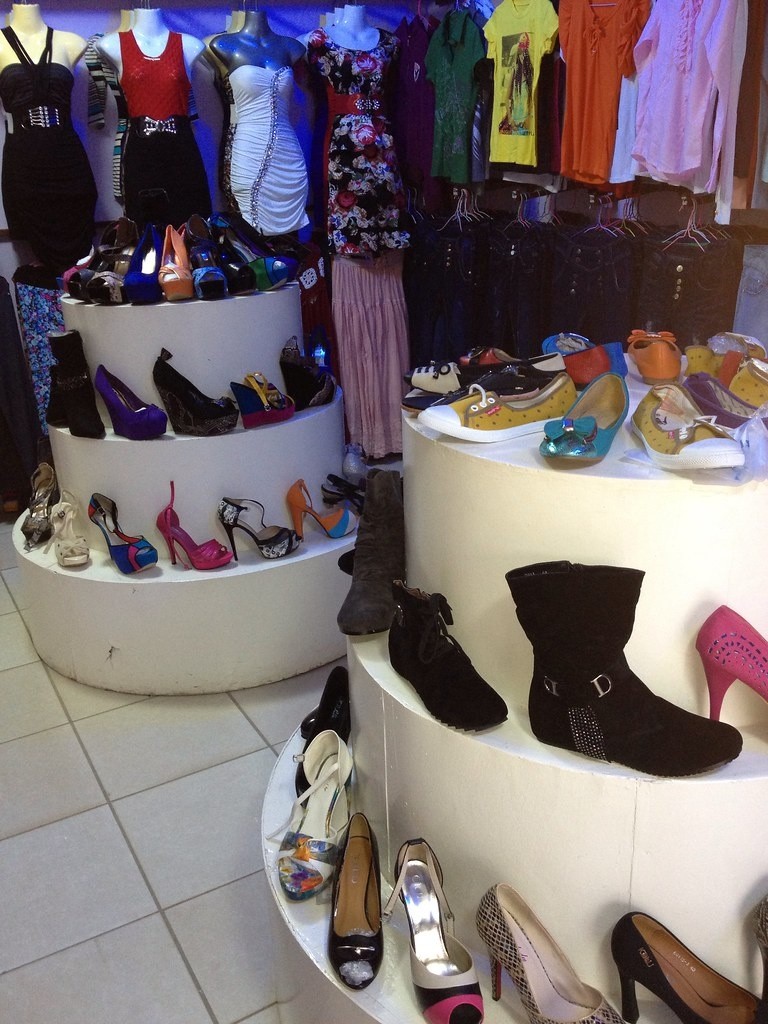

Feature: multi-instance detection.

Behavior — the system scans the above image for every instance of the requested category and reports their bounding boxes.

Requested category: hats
[518,32,530,49]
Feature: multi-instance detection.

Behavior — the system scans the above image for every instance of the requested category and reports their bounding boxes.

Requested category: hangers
[402,186,732,256]
[416,0,430,27]
[449,0,463,15]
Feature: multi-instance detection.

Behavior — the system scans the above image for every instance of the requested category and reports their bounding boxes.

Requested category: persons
[1,1,416,261]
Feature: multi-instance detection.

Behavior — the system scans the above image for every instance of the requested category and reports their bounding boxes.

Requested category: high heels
[278,730,353,904]
[228,372,295,430]
[154,480,234,570]
[280,337,336,412]
[379,838,485,1024]
[95,364,168,440]
[215,496,303,562]
[153,349,240,436]
[694,606,766,721]
[42,489,91,568]
[299,665,349,740]
[327,812,385,992]
[340,446,403,484]
[86,492,157,575]
[286,478,357,543]
[61,211,301,306]
[611,912,763,1024]
[20,462,56,551]
[321,473,364,514]
[474,884,625,1024]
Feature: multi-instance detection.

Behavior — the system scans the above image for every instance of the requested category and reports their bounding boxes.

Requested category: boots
[46,331,108,438]
[335,469,403,635]
[387,581,510,732]
[506,560,744,777]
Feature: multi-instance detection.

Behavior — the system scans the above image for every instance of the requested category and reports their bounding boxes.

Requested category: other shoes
[394,312,767,472]
